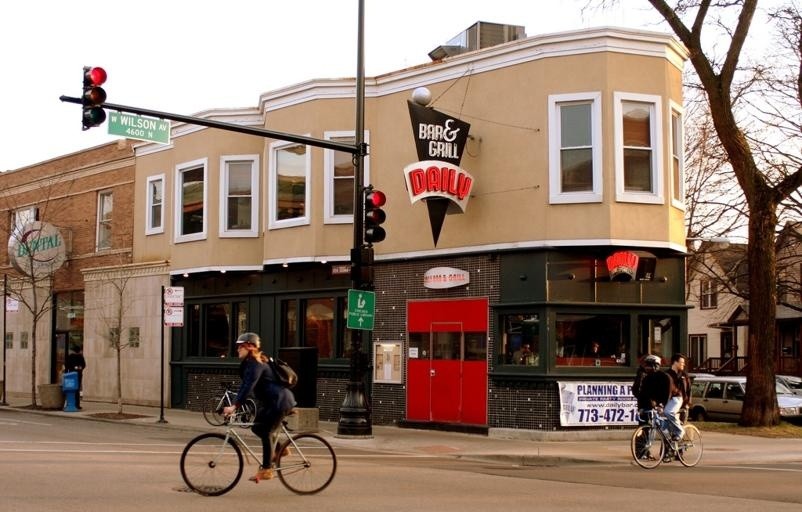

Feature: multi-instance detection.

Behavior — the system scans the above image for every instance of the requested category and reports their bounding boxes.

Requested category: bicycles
[200,382,256,429]
[180,407,337,495]
[630,406,704,469]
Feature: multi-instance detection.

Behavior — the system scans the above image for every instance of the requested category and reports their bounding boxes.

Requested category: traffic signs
[107,113,173,146]
[346,289,375,331]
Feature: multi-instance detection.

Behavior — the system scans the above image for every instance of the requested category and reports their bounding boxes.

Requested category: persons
[65,346,85,409]
[631,353,692,463]
[511,343,531,364]
[223,332,297,481]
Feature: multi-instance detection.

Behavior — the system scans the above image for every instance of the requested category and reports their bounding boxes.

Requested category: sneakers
[248,467,273,481]
[640,453,684,462]
[271,447,291,463]
[674,430,685,440]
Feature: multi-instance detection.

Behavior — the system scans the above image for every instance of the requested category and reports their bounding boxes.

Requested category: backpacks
[262,353,298,388]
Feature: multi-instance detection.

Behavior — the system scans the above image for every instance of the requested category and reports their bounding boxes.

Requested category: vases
[37,384,64,409]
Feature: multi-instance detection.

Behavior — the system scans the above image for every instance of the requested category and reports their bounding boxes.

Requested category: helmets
[643,354,663,365]
[235,331,261,349]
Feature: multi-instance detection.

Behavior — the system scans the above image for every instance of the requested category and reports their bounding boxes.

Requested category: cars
[688,374,802,419]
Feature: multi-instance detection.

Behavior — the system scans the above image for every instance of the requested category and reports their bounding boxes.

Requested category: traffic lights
[363,187,386,244]
[80,64,106,132]
[350,248,375,284]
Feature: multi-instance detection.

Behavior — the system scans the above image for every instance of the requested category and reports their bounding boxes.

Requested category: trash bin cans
[278,345,320,407]
